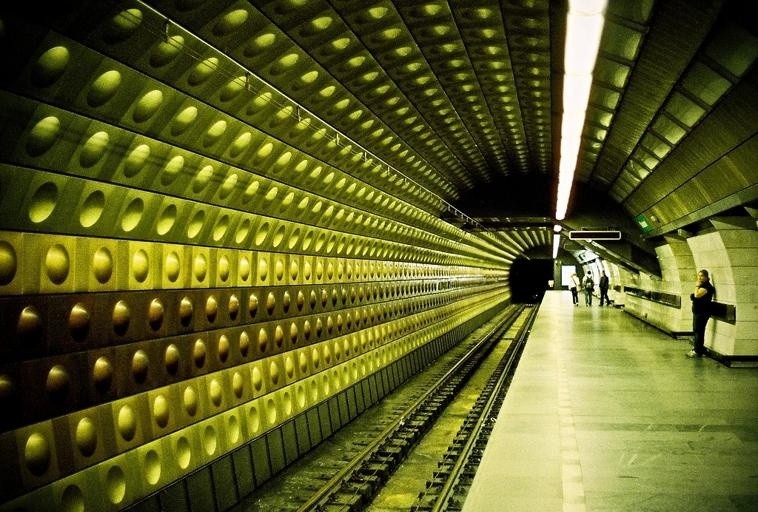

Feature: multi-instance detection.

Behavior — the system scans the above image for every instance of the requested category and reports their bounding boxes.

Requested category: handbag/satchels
[575,284,584,292]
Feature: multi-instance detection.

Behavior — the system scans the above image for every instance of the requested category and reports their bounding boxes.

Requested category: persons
[684,268,715,357]
[599,270,610,305]
[581,271,597,306]
[568,272,580,307]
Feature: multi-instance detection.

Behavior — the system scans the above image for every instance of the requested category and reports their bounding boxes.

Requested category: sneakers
[686,351,704,358]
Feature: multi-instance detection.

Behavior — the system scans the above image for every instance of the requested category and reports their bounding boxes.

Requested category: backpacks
[585,276,593,291]
